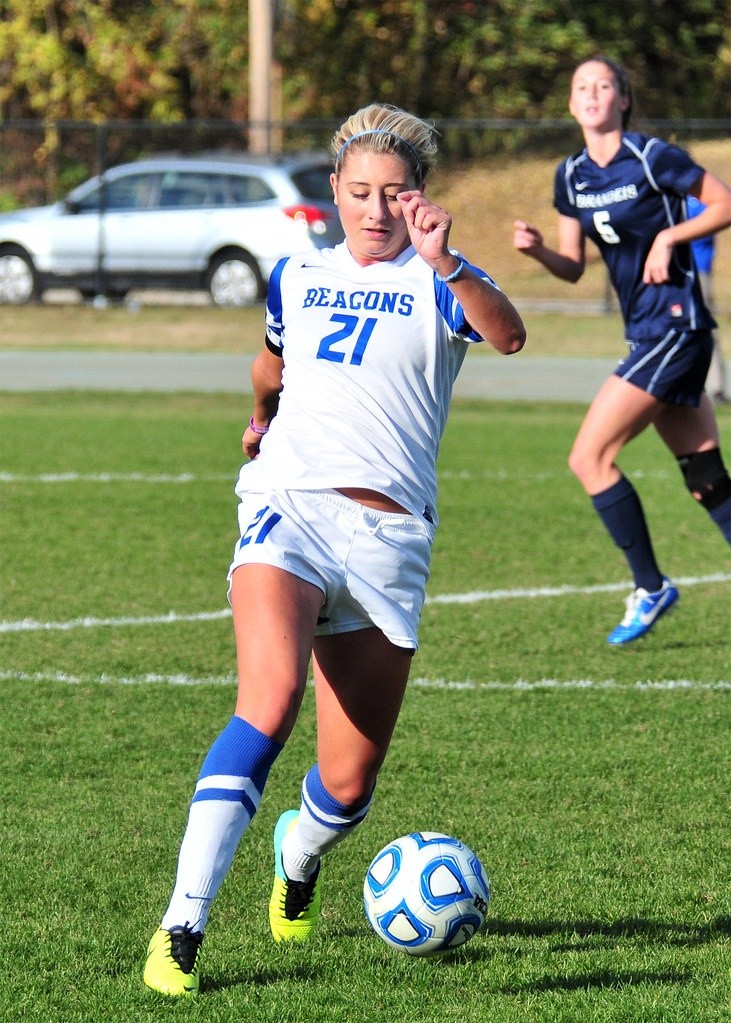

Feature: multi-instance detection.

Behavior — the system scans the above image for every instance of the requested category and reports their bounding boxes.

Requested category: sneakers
[143,919,204,998]
[269,810,320,945]
[609,574,678,646]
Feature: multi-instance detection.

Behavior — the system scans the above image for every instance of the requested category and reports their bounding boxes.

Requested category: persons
[512,55,731,647]
[144,103,528,997]
[683,192,731,401]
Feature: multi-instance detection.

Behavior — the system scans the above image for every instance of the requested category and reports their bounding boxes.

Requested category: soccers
[362,830,492,957]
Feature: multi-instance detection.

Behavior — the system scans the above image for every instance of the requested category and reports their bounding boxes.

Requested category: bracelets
[249,416,269,433]
[436,257,463,282]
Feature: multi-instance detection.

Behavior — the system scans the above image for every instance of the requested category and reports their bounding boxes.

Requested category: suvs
[0,150,344,309]
